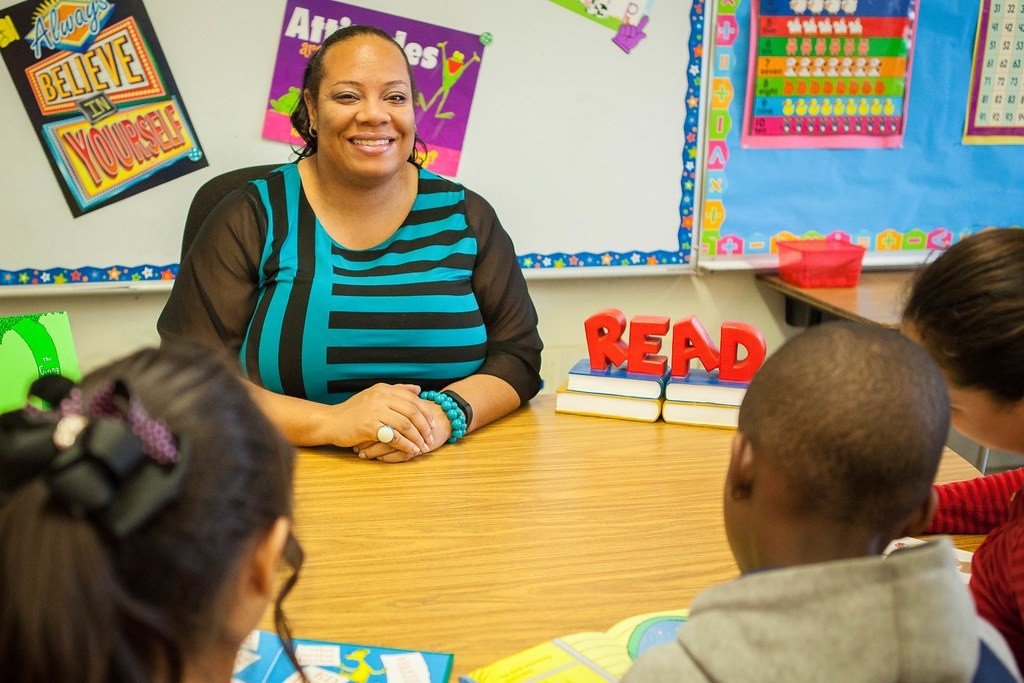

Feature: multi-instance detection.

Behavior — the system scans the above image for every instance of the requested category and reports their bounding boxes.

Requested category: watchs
[439,390,474,430]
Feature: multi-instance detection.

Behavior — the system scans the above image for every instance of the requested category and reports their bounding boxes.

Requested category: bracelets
[419,390,467,444]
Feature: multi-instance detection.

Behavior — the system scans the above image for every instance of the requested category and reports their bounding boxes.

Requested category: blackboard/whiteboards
[0,1,709,301]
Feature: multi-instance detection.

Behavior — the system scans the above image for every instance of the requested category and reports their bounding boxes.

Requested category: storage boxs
[776,239,869,288]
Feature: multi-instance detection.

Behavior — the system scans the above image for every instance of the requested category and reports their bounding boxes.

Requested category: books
[227,628,456,683]
[457,608,692,683]
[554,359,752,431]
[883,537,974,586]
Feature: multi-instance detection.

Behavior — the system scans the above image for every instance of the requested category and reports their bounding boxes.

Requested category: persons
[155,26,544,463]
[910,228,1024,683]
[615,320,982,683]
[1,346,312,683]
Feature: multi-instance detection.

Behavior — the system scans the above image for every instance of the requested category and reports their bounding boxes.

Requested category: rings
[377,426,394,444]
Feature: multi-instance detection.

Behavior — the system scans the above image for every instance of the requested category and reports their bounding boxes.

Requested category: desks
[755,263,1024,479]
[253,393,997,683]
